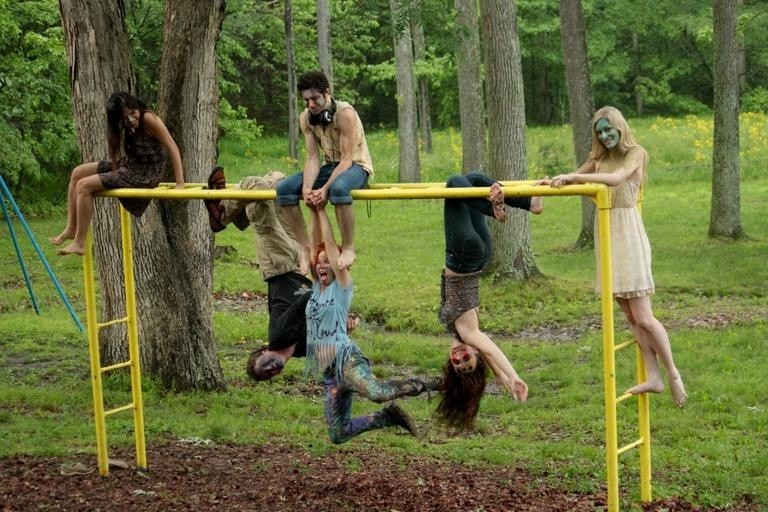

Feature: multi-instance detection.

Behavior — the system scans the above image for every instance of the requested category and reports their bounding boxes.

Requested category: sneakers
[385,404,417,436]
[208,167,226,203]
[417,374,445,391]
[202,186,227,232]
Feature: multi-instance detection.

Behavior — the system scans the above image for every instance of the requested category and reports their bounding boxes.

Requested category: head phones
[306,94,337,125]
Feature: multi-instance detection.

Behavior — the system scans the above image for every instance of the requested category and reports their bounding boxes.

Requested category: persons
[275,71,374,271]
[46,92,186,255]
[531,105,689,409]
[202,164,356,381]
[430,169,551,429]
[301,187,442,446]
